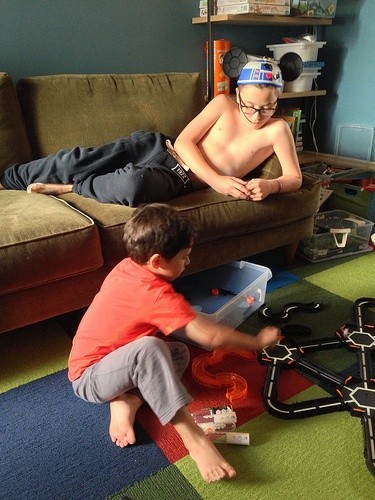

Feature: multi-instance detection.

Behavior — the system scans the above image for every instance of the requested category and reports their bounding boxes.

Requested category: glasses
[238,91,279,116]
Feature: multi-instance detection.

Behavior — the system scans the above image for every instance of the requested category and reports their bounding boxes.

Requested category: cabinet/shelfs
[192,0,338,152]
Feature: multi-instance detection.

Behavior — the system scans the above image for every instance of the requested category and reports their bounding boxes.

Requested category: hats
[236,59,285,89]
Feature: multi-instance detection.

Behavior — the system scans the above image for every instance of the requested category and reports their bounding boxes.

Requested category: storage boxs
[297,209,374,260]
[266,42,326,93]
[164,259,273,351]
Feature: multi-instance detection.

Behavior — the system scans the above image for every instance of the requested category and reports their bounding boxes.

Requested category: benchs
[0,73,322,334]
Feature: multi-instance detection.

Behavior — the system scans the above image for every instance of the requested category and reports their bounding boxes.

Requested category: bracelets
[273,179,282,193]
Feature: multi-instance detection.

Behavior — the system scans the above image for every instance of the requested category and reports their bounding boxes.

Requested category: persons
[0,58,301,206]
[67,203,282,483]
